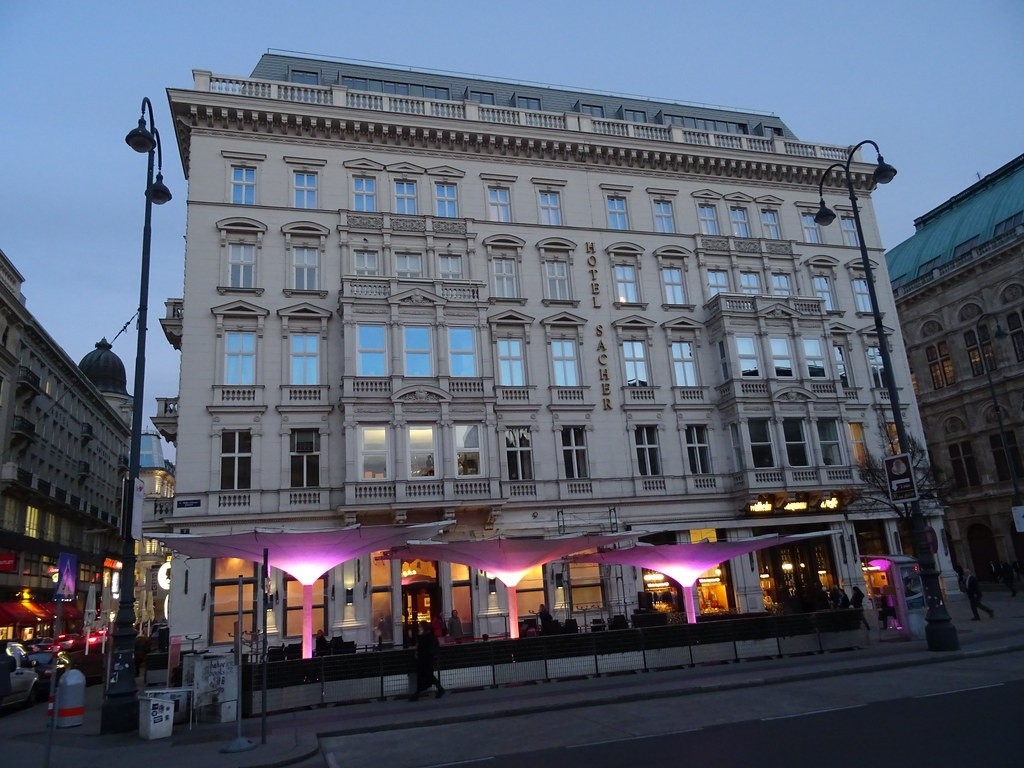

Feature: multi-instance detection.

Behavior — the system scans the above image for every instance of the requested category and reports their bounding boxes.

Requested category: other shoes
[408,694,419,701]
[972,617,980,621]
[1012,591,1017,597]
[867,627,871,631]
[436,690,445,699]
[990,609,994,618]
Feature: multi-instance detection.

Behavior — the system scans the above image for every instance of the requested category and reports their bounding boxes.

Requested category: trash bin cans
[54,669,86,728]
[138,695,175,740]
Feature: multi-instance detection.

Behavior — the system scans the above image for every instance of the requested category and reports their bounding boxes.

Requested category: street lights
[101,95,174,732]
[812,138,961,653]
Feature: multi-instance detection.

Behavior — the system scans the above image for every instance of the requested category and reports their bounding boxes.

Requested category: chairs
[519,614,629,636]
[266,636,357,662]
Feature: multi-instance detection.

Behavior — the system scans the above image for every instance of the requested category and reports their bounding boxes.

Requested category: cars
[23,629,108,654]
[27,649,73,691]
[149,624,166,636]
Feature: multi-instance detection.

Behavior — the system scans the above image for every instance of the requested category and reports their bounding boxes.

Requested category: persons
[879,585,898,629]
[447,610,464,644]
[820,586,870,631]
[952,564,994,621]
[316,630,330,657]
[408,620,445,701]
[990,560,1024,597]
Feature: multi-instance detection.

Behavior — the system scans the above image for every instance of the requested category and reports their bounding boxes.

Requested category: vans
[0,641,42,710]
